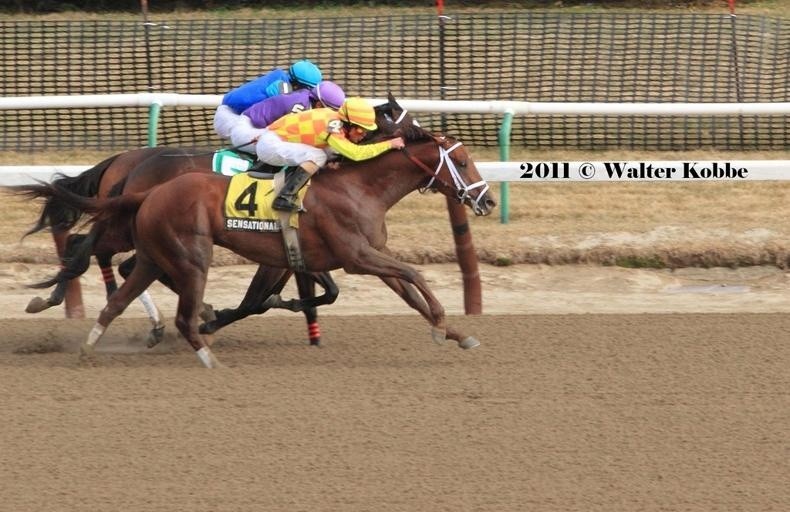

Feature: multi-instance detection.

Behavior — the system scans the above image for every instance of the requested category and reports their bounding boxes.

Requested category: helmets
[337,98,379,132]
[311,81,346,110]
[289,60,322,87]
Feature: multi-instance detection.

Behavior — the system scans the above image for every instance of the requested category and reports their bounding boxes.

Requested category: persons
[254,95,407,212]
[212,60,323,141]
[230,80,345,156]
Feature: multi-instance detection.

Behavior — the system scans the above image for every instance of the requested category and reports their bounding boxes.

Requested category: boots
[272,166,310,212]
[247,161,271,174]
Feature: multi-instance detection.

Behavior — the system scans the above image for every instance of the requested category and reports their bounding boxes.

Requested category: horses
[0,88,496,373]
